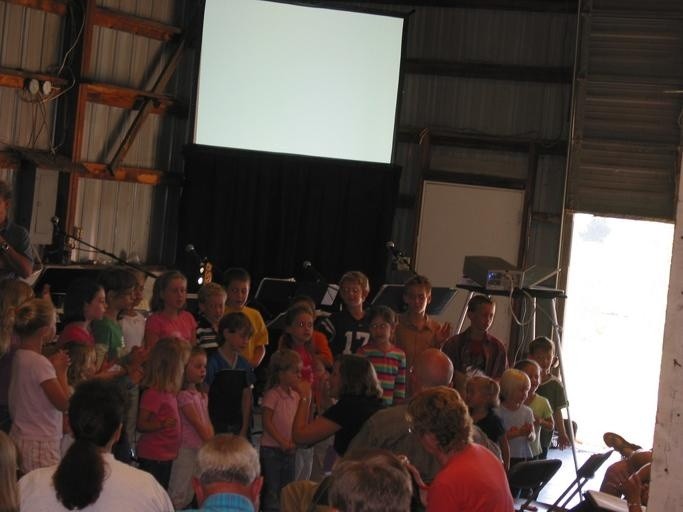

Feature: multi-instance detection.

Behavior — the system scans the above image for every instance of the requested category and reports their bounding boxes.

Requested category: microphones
[303,260,322,281]
[51,216,61,232]
[386,241,399,260]
[185,244,205,267]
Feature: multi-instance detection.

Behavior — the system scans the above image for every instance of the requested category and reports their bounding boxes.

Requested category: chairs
[507,458,562,512]
[547,449,615,511]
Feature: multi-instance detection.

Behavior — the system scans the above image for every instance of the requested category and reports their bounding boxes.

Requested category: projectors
[463,256,525,291]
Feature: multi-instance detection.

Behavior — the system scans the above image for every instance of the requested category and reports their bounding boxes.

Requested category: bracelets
[1,240,10,251]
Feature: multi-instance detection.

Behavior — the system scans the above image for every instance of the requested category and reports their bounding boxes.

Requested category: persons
[0,179,35,280]
[600,432,654,507]
[2,264,571,511]
